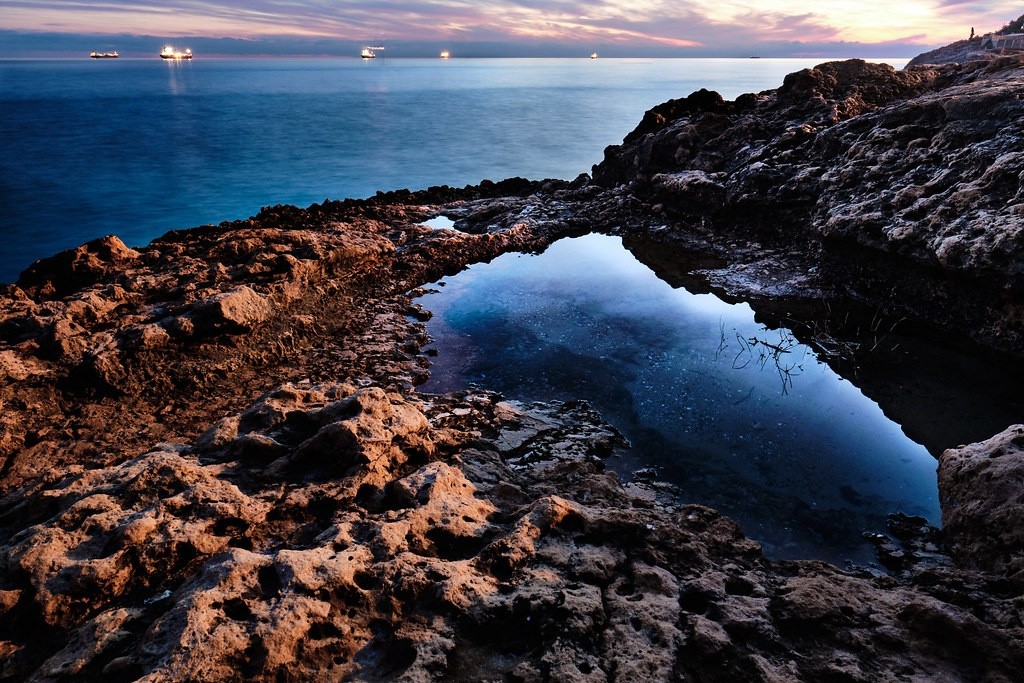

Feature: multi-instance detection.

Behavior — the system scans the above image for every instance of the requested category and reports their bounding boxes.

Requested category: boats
[89,52,118,58]
[359,49,375,58]
[440,53,447,59]
[590,53,597,59]
[160,48,193,59]
